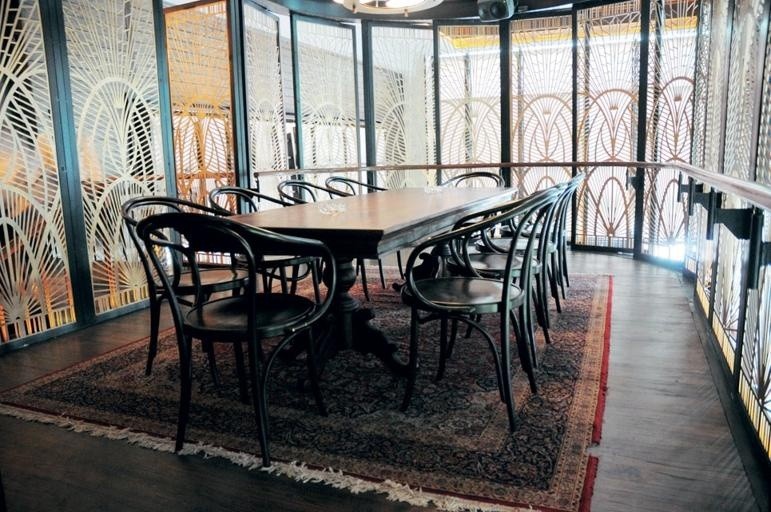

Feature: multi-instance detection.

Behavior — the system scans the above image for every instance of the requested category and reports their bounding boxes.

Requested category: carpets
[3,264,615,511]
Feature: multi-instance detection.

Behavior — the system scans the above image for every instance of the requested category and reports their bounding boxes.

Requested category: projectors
[476,0,515,23]
